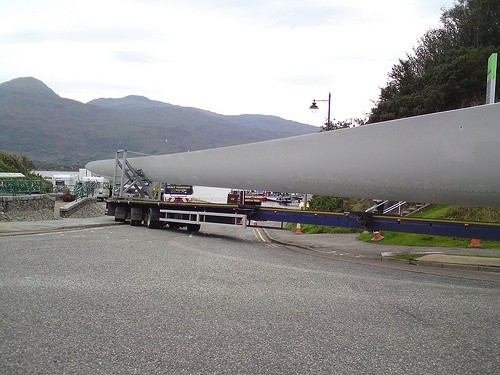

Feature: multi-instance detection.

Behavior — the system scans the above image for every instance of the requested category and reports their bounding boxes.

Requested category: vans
[52,175,79,196]
[82,177,110,203]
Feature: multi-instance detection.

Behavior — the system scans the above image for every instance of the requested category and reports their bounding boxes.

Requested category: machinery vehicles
[83,103,500,241]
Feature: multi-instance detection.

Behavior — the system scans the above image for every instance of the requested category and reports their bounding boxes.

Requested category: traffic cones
[294,223,304,236]
[371,231,384,241]
[470,238,482,248]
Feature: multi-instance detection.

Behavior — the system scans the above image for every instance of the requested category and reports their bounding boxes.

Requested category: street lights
[309,93,332,131]
